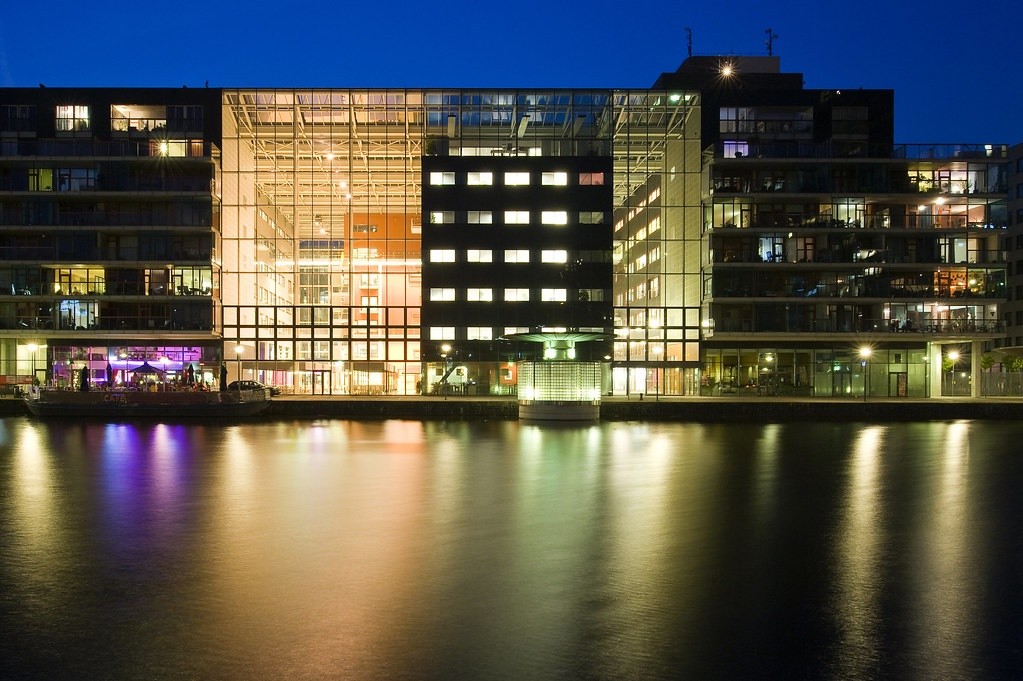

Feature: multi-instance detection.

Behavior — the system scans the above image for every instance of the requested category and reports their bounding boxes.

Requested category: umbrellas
[187,364,195,387]
[219,365,229,391]
[79,366,90,391]
[126,361,167,391]
[106,363,114,387]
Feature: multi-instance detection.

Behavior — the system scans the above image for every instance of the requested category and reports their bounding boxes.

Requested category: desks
[189,288,202,296]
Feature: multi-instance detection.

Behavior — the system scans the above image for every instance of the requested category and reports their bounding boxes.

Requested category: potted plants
[957,177,972,194]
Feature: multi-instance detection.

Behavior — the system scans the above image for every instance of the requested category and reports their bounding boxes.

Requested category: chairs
[202,286,210,296]
[455,386,459,395]
[463,382,468,395]
[177,286,181,295]
[183,286,193,296]
